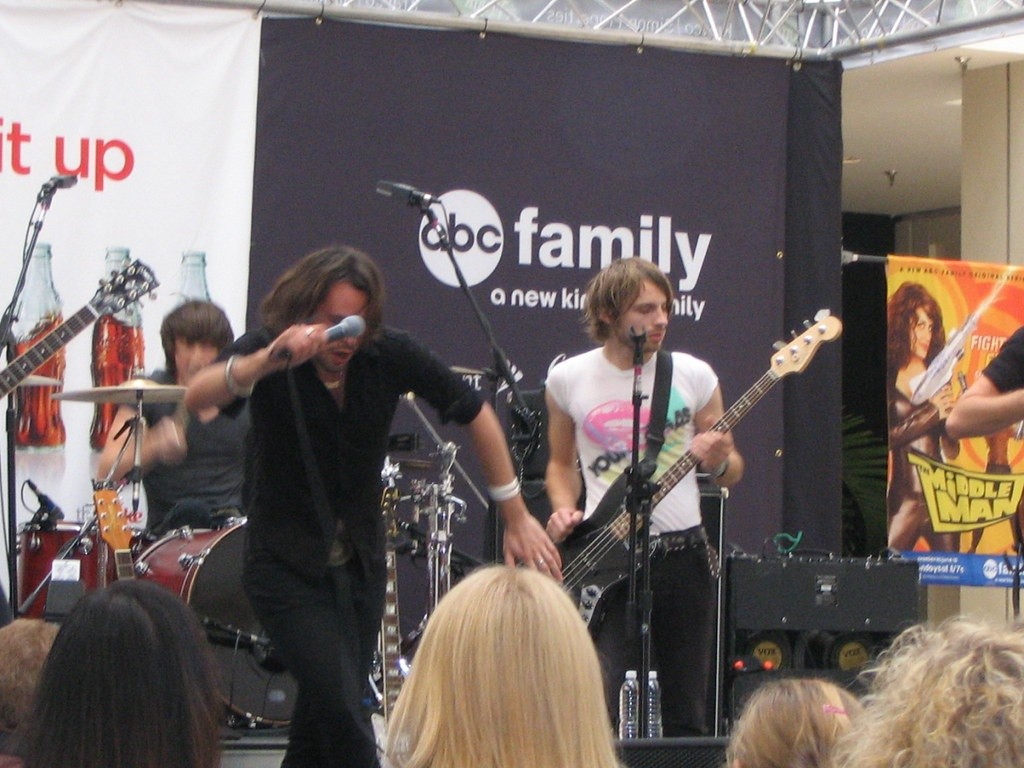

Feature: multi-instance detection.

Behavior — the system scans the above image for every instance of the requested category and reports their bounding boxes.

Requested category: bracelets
[225,353,255,400]
[710,457,731,481]
[483,475,523,503]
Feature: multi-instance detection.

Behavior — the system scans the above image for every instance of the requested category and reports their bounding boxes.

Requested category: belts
[663,527,707,549]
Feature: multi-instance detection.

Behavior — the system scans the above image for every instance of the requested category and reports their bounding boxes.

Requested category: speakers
[487,390,921,768]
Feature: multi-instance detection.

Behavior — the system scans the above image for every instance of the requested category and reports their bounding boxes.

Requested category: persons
[944,326,1024,441]
[0,577,222,768]
[887,281,960,552]
[544,257,744,738]
[185,245,564,768]
[967,426,1024,554]
[94,298,250,535]
[829,614,1024,768]
[379,563,621,768]
[724,677,864,768]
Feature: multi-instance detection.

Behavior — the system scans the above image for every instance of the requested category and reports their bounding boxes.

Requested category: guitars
[518,309,844,643]
[93,478,137,581]
[0,259,161,397]
[370,485,416,768]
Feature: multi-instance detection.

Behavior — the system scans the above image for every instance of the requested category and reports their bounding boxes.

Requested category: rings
[304,326,316,337]
[536,558,545,565]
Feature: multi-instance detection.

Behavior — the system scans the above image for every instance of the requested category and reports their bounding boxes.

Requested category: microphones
[390,183,441,208]
[42,174,78,193]
[281,315,366,359]
[28,480,64,520]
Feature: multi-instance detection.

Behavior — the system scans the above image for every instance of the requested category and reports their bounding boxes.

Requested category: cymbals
[400,365,487,375]
[18,375,190,403]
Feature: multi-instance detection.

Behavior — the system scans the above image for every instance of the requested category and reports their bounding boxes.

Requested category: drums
[15,520,145,631]
[131,520,301,728]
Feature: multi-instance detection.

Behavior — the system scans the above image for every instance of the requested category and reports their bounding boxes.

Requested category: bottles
[90,249,145,453]
[12,245,67,450]
[648,671,663,738]
[619,671,639,741]
[178,254,212,304]
